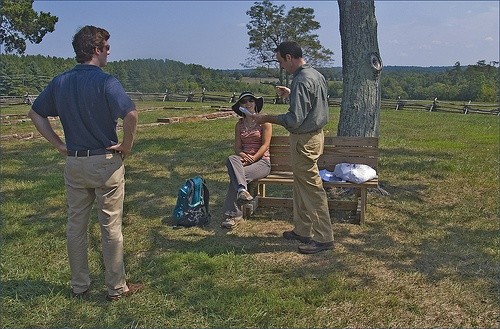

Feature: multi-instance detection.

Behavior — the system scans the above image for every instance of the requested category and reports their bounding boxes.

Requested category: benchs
[243,135,379,226]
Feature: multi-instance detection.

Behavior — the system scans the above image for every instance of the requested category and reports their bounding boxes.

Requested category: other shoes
[106,284,144,302]
[283,229,312,244]
[221,214,243,229]
[237,189,253,201]
[298,239,335,254]
[72,292,86,298]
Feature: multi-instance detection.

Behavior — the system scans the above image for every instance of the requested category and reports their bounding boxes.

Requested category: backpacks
[173,176,211,228]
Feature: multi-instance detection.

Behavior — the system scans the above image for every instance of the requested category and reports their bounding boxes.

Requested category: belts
[66,149,116,157]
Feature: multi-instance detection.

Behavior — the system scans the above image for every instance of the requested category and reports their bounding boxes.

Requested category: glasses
[104,44,110,50]
[243,98,254,103]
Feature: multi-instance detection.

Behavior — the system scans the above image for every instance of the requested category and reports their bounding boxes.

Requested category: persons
[253,42,334,255]
[28,25,144,302]
[221,92,272,230]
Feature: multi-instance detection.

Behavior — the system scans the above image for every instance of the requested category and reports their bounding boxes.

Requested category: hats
[231,91,264,117]
